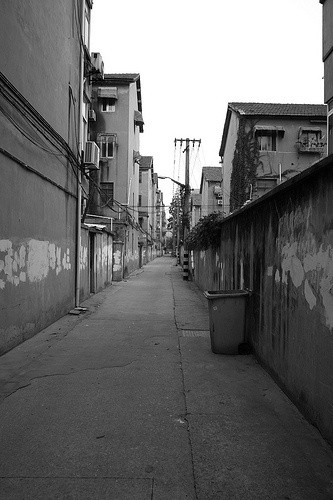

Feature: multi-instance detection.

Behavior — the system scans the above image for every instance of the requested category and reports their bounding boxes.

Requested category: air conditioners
[88,109,98,123]
[83,140,101,171]
[217,200,222,205]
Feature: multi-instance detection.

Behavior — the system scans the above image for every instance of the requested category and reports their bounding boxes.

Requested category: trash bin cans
[203,286,254,355]
